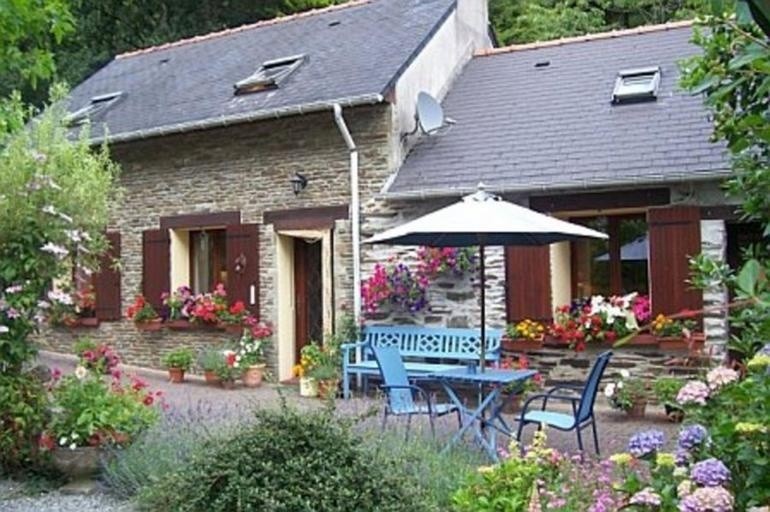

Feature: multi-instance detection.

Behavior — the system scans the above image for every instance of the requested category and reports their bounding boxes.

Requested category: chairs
[370,345,466,448]
[513,350,613,467]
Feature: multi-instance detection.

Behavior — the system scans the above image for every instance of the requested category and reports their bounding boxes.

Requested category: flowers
[289,341,323,379]
[358,245,477,325]
[504,291,699,411]
[38,286,273,448]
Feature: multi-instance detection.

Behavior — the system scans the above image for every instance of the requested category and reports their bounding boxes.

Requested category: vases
[298,375,318,397]
[624,401,647,418]
[51,446,124,479]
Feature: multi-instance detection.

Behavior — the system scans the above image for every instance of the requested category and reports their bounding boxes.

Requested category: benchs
[340,326,505,412]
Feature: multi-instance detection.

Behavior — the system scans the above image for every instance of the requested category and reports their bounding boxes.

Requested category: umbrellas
[594,236,648,261]
[364,187,609,433]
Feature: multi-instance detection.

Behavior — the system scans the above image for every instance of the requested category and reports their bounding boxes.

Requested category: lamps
[291,172,307,197]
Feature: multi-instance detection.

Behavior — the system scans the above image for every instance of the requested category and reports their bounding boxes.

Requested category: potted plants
[314,315,361,400]
[652,378,689,423]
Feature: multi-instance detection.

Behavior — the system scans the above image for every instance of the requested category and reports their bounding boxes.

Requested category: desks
[428,368,539,465]
